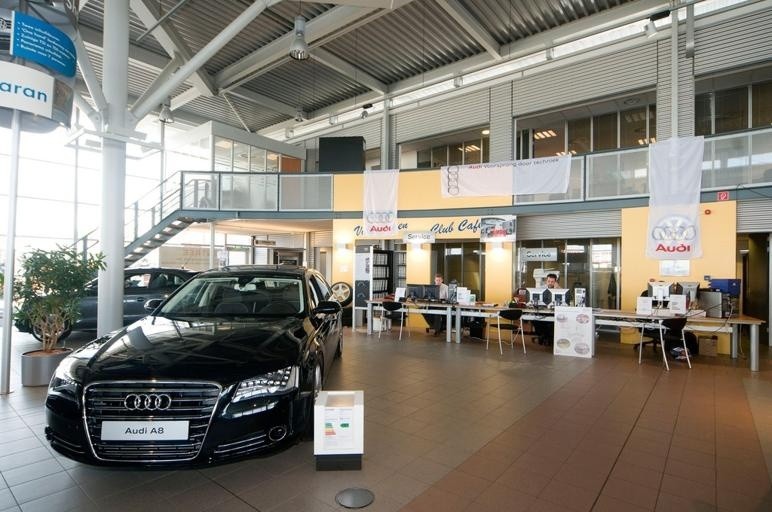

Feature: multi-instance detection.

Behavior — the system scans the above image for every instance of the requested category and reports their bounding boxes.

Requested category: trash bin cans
[698,336,717,356]
[470,321,486,341]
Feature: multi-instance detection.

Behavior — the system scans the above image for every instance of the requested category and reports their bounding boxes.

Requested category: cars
[15,268,197,343]
[43,265,344,472]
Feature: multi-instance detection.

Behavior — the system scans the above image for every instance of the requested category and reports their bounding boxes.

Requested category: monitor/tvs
[676,282,700,309]
[526,287,549,310]
[547,288,570,309]
[422,285,440,302]
[406,284,424,301]
[648,282,674,309]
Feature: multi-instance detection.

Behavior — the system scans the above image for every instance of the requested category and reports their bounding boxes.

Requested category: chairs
[124,274,182,289]
[485,308,527,355]
[531,320,555,347]
[424,319,446,338]
[633,290,672,354]
[378,301,406,341]
[635,315,692,372]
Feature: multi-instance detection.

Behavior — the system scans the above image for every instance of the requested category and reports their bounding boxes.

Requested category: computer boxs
[700,290,732,318]
[574,288,588,308]
[448,283,459,303]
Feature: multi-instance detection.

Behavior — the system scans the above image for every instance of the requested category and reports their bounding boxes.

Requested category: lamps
[159,96,174,124]
[640,10,674,37]
[294,83,304,122]
[288,0,312,61]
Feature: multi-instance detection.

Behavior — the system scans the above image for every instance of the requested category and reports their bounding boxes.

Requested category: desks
[365,295,765,372]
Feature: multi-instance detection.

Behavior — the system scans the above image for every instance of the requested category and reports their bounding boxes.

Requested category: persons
[535,274,562,345]
[432,273,449,336]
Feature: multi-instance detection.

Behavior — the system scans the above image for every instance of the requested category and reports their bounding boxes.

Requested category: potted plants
[0,247,108,388]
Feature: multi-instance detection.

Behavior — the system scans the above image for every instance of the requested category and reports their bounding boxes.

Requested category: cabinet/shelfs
[212,285,299,312]
